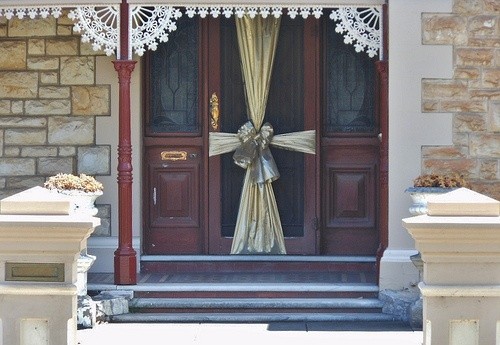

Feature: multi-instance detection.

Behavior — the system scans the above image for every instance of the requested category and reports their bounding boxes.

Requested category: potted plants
[44,172,104,208]
[405,173,462,207]
